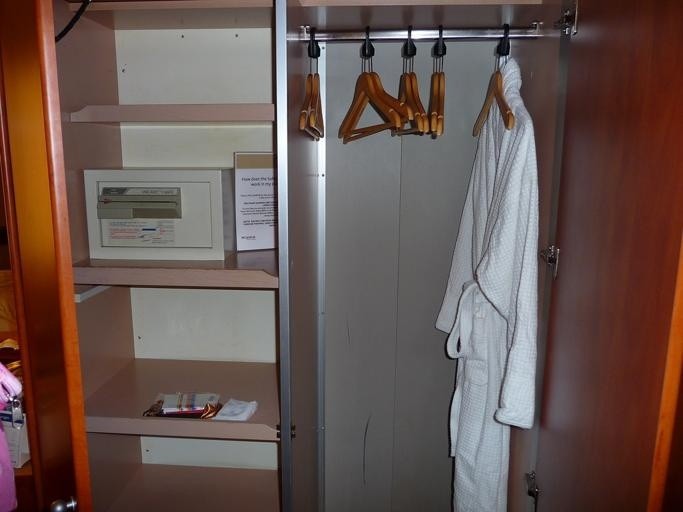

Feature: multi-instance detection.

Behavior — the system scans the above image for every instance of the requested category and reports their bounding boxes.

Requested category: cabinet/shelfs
[32,3,683,511]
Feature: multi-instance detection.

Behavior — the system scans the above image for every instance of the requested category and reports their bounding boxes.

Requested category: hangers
[297,26,514,140]
